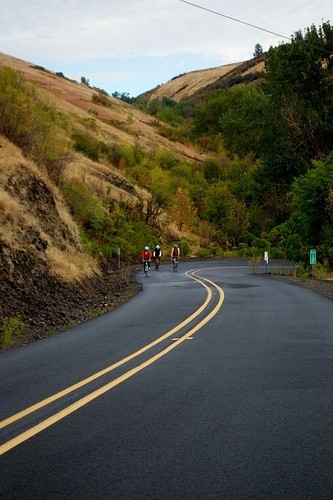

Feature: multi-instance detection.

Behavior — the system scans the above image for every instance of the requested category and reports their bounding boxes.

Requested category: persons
[140,246,152,272]
[151,244,162,266]
[170,244,181,266]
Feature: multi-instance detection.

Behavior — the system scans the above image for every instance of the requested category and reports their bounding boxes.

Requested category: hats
[144,246,149,251]
[155,245,160,249]
[174,244,177,247]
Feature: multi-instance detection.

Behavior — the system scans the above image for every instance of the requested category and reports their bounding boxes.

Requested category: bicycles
[142,259,152,278]
[172,256,179,272]
[154,256,161,272]
